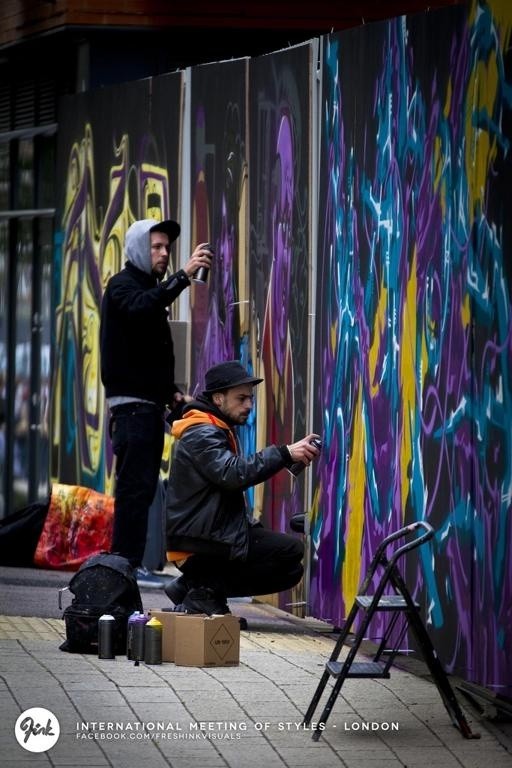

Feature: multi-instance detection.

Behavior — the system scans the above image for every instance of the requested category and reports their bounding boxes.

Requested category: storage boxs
[148,611,240,668]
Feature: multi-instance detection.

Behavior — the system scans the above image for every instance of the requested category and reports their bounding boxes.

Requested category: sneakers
[133,566,175,590]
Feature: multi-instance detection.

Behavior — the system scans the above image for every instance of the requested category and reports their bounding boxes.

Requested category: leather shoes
[163,574,201,614]
[182,584,248,631]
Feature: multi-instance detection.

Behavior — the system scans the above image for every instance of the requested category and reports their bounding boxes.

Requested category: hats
[150,219,182,243]
[201,359,264,395]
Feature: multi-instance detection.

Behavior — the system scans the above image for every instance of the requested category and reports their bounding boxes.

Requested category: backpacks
[0,493,51,567]
[57,550,143,655]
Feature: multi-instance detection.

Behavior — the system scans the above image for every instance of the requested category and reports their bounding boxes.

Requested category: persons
[97,217,216,592]
[164,358,327,635]
